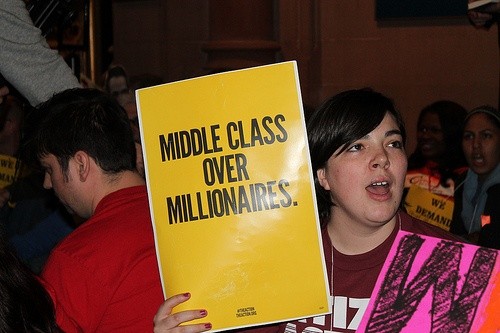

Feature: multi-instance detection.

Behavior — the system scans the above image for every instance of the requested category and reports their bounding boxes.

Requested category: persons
[414,101,500,248]
[136,86,494,332]
[0,0,92,101]
[17,85,203,332]
